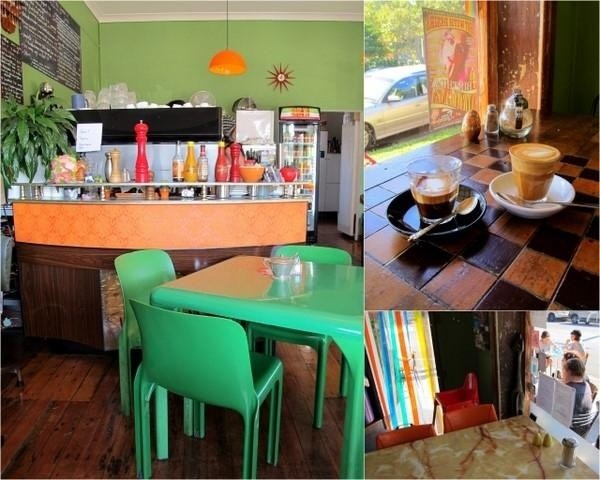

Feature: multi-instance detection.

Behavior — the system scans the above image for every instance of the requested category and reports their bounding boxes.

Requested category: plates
[189,90,217,107]
[488,170,576,220]
[386,183,486,236]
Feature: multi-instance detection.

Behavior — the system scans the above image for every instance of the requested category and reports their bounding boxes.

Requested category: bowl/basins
[236,164,267,183]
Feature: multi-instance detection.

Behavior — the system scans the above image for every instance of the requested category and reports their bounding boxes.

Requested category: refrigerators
[273,104,321,242]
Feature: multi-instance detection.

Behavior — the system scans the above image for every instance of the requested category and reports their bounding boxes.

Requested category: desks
[361,414,599,479]
[148,256,364,479]
[364,119,600,309]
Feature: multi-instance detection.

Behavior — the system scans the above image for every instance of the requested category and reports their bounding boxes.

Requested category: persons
[529,358,593,435]
[562,353,576,366]
[564,330,585,362]
[440,31,456,81]
[530,327,542,360]
[537,330,556,376]
[446,31,470,87]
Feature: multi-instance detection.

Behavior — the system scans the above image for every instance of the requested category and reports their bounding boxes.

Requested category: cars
[546,310,600,328]
[364,63,429,151]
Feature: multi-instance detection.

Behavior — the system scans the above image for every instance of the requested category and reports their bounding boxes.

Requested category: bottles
[561,436,578,468]
[300,171,312,181]
[304,196,312,208]
[170,136,241,183]
[103,147,122,183]
[131,119,151,181]
[281,145,315,157]
[283,158,313,168]
[282,130,314,144]
[483,103,500,136]
[302,184,315,194]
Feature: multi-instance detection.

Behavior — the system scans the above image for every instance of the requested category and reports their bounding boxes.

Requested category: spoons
[496,190,600,213]
[406,196,479,246]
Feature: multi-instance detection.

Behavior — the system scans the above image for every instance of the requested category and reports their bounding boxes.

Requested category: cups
[101,187,169,202]
[509,143,563,202]
[70,93,90,109]
[198,185,261,200]
[19,184,100,201]
[406,154,463,225]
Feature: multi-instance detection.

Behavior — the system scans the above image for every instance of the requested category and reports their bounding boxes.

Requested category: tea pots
[262,251,301,281]
[500,87,535,139]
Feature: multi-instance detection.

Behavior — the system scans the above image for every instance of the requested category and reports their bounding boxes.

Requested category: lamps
[209,2,246,76]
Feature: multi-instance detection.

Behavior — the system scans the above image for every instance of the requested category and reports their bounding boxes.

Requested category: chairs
[436,372,478,418]
[251,244,354,428]
[0,234,25,389]
[115,249,195,436]
[125,294,284,480]
[444,404,500,432]
[378,423,436,453]
